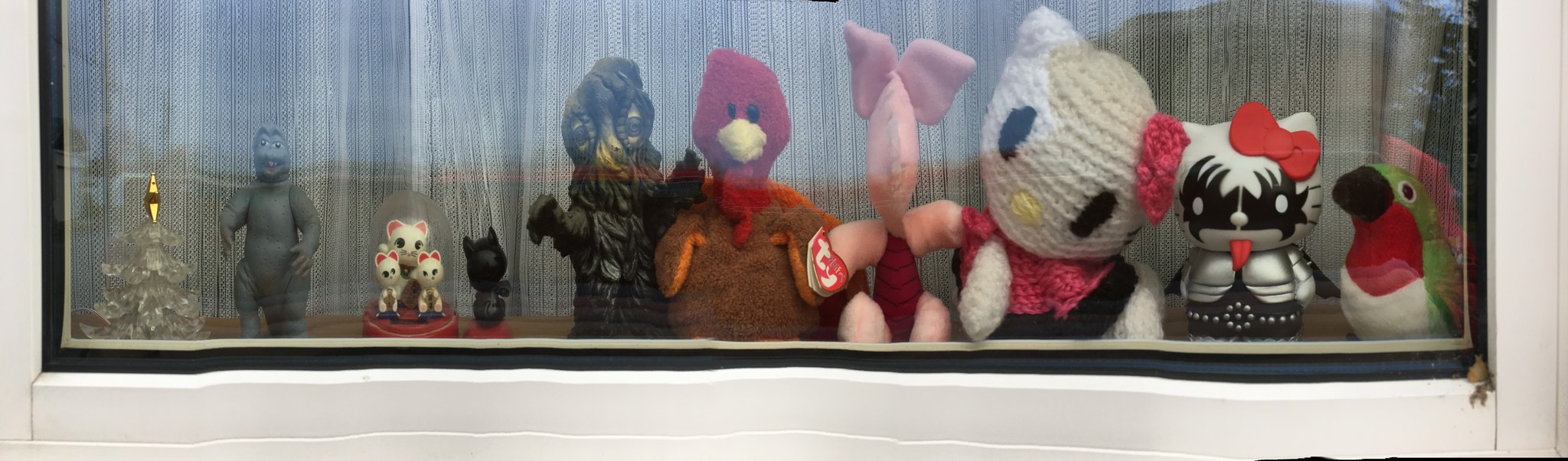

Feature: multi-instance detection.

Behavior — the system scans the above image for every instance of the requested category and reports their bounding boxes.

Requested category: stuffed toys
[650,47,873,344]
[1331,163,1467,344]
[953,1,1194,341]
[803,18,977,343]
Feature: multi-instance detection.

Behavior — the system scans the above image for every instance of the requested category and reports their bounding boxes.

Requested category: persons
[217,125,321,339]
